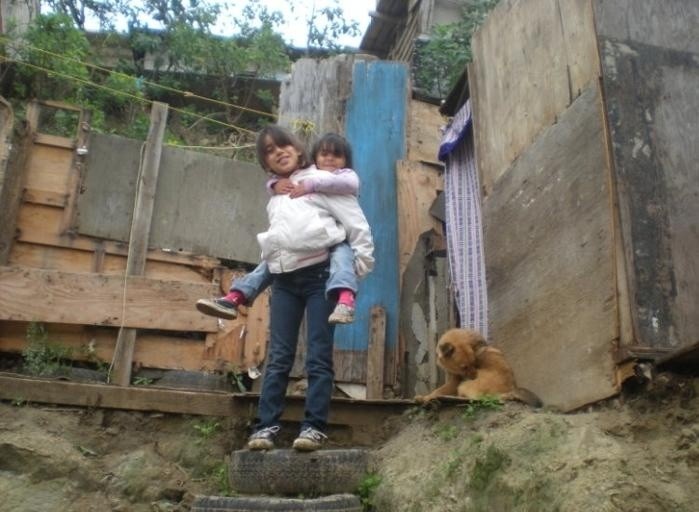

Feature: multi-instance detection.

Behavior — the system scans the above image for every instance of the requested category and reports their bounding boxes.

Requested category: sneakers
[293,427,328,450]
[196,297,238,320]
[247,425,280,450]
[328,303,355,324]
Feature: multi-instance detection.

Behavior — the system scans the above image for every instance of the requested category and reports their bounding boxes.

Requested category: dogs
[413,327,545,410]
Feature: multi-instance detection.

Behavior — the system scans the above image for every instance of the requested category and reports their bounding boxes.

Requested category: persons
[246,124,375,452]
[194,132,358,323]
